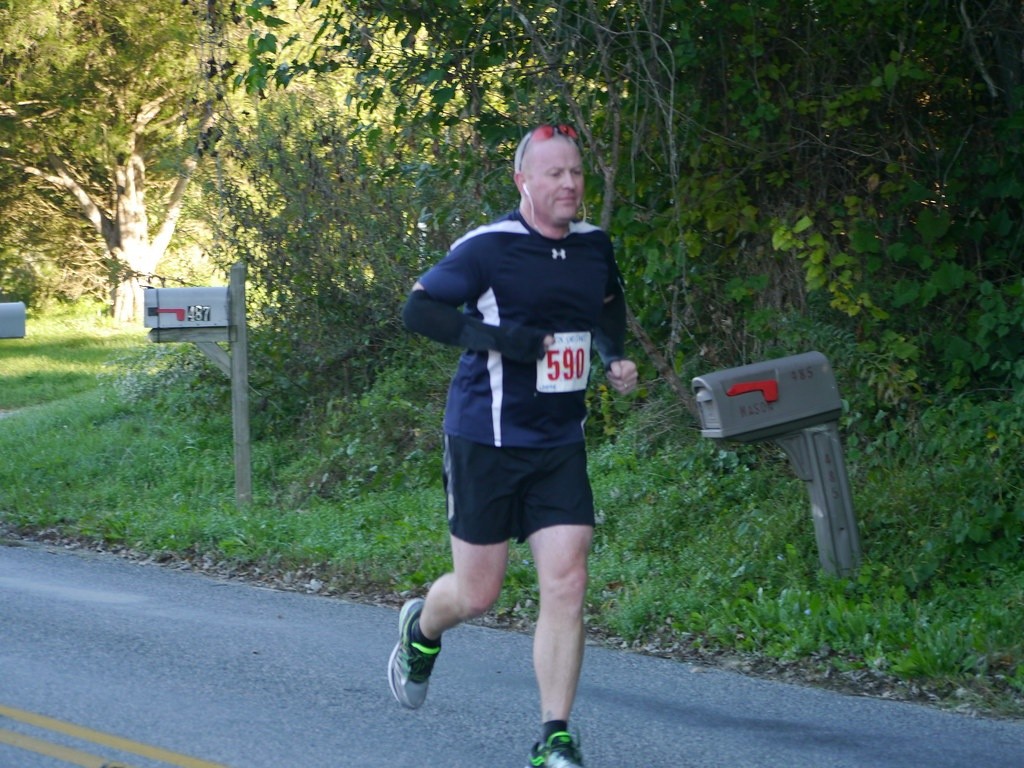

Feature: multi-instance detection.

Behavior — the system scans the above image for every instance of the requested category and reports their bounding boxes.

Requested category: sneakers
[528,731,586,768]
[388,598,442,708]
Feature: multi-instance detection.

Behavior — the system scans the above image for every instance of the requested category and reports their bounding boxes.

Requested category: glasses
[519,124,578,170]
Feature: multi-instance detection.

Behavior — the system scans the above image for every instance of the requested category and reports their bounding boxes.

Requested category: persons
[387,124,639,768]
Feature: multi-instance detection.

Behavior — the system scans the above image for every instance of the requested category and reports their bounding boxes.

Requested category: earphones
[523,182,530,198]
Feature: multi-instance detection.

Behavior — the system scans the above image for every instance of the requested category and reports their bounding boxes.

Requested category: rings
[624,384,628,389]
